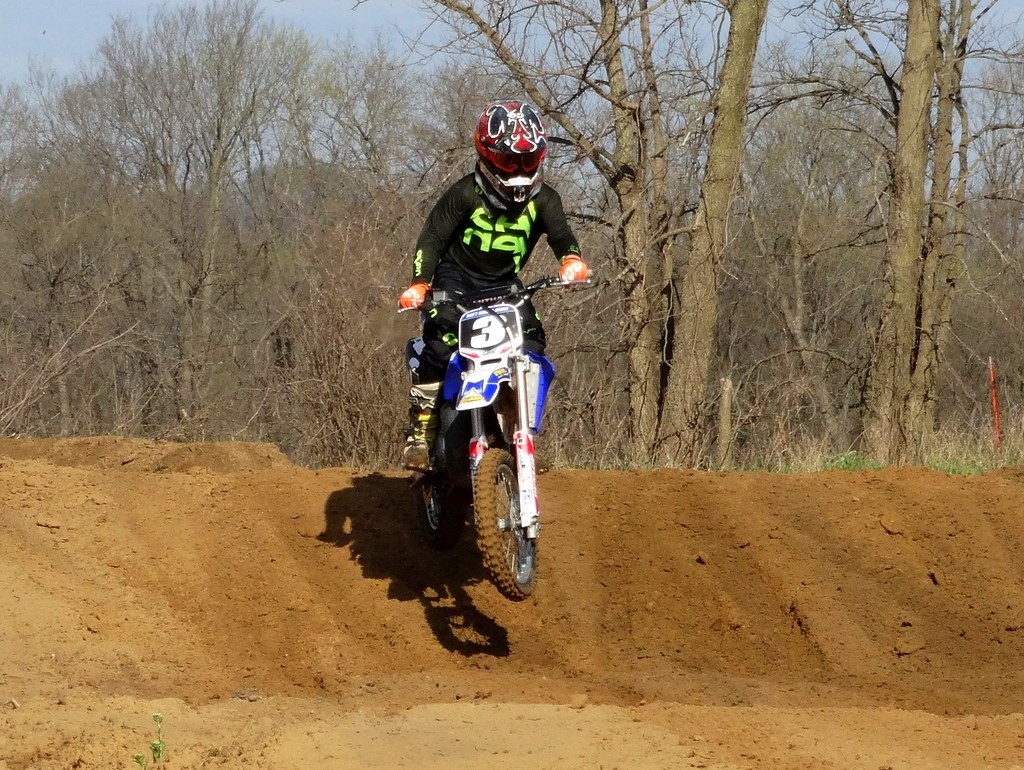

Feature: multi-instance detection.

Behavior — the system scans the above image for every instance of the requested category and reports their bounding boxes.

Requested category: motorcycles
[396,268,596,603]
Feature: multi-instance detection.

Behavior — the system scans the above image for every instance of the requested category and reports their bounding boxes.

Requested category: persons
[399,101,590,474]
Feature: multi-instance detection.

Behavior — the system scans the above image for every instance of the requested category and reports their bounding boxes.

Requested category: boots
[403,382,442,467]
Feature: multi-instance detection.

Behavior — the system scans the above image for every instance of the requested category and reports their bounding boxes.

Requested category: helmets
[474,100,547,217]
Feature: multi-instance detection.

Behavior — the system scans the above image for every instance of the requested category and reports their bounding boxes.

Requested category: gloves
[559,253,589,290]
[399,282,428,310]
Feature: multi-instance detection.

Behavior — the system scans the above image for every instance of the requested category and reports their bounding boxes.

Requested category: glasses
[493,149,544,172]
